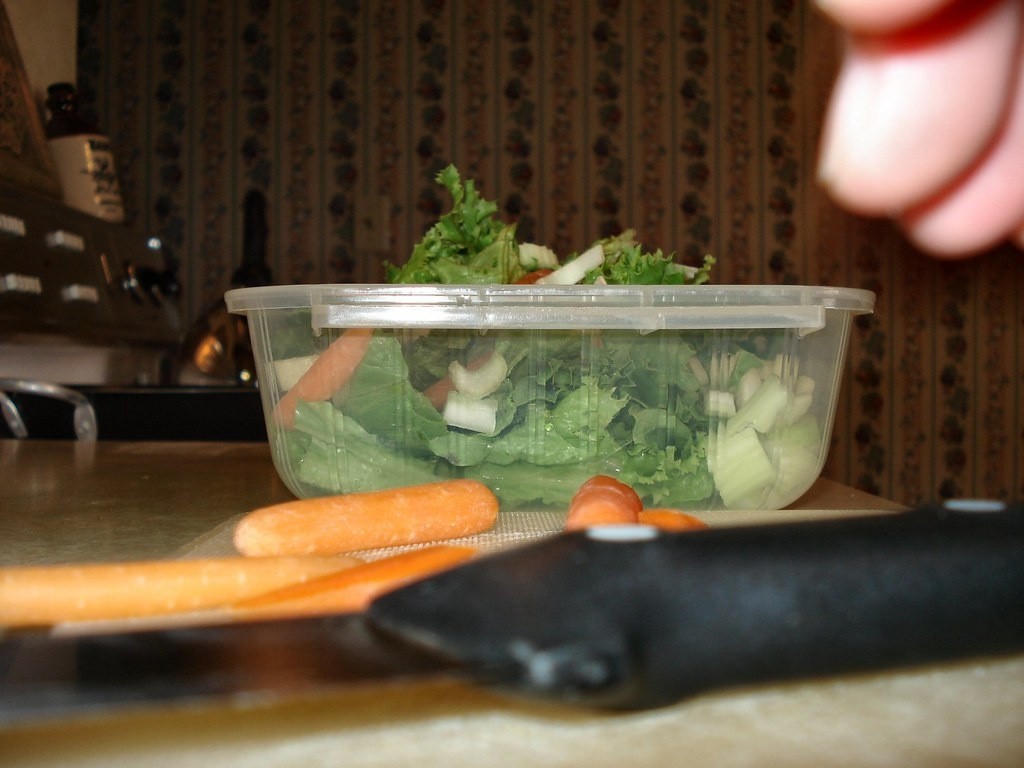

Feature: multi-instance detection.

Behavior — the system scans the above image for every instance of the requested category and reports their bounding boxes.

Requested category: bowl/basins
[223,285,876,511]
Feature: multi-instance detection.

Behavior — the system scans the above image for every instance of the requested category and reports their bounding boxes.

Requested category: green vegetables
[272,161,824,512]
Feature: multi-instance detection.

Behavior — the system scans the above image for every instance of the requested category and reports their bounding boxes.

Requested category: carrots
[0,479,499,628]
[569,476,704,530]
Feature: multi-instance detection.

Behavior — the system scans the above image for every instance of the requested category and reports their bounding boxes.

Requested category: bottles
[232,189,273,389]
[97,254,187,344]
[44,81,126,225]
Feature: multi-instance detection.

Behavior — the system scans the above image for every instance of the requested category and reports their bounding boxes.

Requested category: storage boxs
[230,280,878,511]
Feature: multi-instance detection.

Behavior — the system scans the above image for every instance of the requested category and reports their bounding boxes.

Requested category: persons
[810,0,1024,259]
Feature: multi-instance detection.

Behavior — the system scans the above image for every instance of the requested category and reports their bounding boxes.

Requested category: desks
[1,432,914,698]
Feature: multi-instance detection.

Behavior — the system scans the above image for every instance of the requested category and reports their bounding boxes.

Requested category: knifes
[0,496,1024,729]
